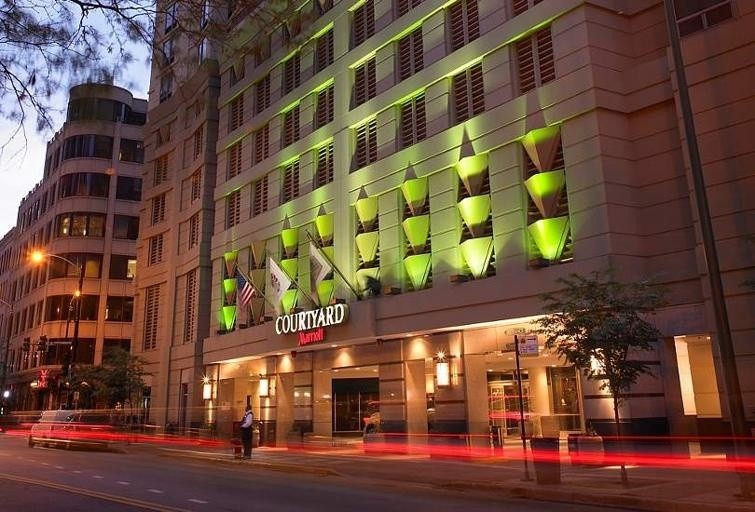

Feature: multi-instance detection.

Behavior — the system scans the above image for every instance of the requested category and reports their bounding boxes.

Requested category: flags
[235,269,255,326]
[307,240,331,309]
[264,257,291,319]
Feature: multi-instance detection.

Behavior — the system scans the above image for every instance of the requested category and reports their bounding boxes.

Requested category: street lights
[31,250,86,409]
[4,391,9,429]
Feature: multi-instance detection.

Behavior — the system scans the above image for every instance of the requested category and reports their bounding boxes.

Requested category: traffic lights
[22,338,30,352]
[38,335,48,350]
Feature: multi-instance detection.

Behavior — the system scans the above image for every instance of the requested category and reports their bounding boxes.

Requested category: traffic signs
[518,335,538,355]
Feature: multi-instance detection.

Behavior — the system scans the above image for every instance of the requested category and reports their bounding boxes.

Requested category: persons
[240,404,253,460]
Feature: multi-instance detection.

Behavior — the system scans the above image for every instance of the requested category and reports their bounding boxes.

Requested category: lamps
[259,378,270,398]
[202,384,212,400]
[436,361,451,387]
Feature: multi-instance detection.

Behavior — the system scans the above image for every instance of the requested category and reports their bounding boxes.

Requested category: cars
[27,412,96,450]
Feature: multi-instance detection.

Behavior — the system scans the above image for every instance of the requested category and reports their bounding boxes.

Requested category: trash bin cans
[529,437,561,485]
